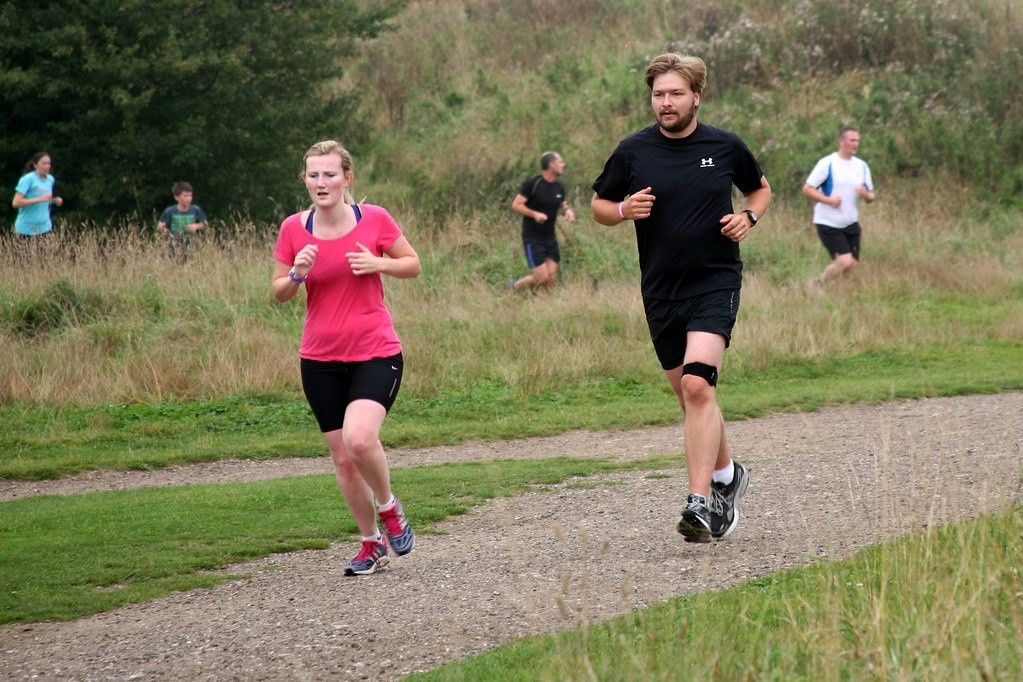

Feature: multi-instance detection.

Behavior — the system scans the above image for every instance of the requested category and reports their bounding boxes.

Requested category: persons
[272,140,424,577]
[801,125,875,284]
[12,152,62,239]
[591,52,772,544]
[504,150,575,295]
[157,181,208,264]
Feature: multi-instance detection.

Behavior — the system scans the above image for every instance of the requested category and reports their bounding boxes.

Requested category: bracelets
[619,200,629,220]
[562,207,569,215]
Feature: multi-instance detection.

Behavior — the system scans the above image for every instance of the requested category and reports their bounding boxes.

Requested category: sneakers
[378,498,415,556]
[710,463,750,539]
[344,536,390,576]
[677,494,713,545]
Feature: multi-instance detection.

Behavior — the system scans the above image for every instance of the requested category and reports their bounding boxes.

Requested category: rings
[738,229,743,235]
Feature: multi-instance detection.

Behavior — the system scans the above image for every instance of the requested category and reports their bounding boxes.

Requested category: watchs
[741,209,757,229]
[288,265,307,284]
